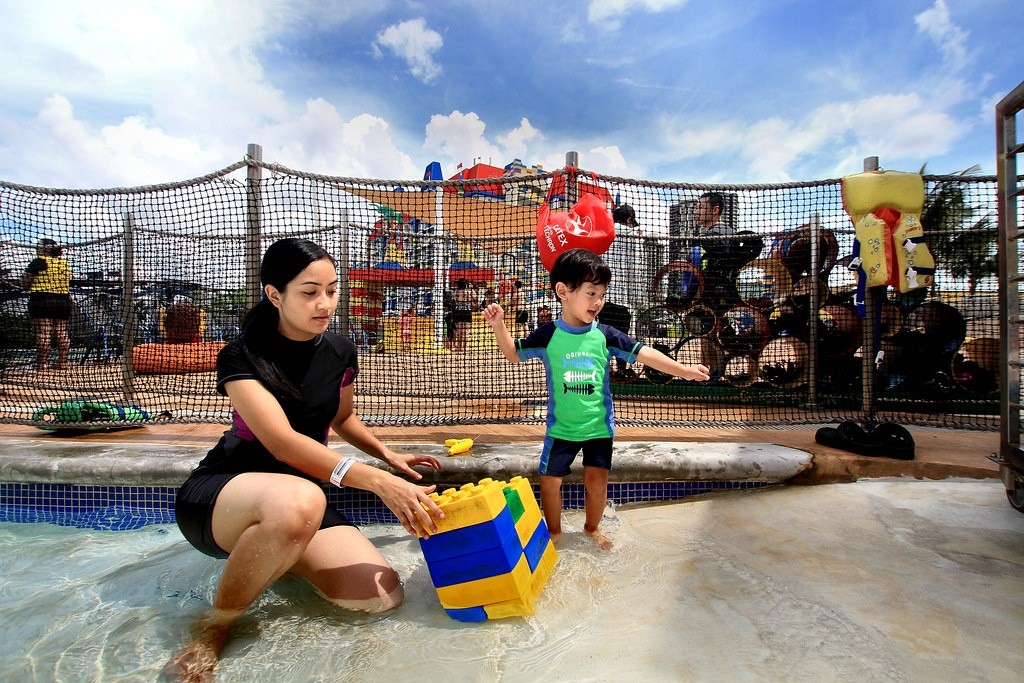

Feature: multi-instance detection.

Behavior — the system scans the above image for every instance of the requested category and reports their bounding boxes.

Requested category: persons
[19,238,71,369]
[481,248,710,534]
[694,193,735,372]
[444,278,477,355]
[397,310,412,354]
[513,281,528,339]
[175,238,445,608]
[537,305,550,324]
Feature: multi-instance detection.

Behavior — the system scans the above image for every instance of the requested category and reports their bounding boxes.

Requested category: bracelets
[330,456,355,488]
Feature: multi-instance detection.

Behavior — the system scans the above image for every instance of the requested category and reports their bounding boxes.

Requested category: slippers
[815,421,877,455]
[872,422,919,460]
